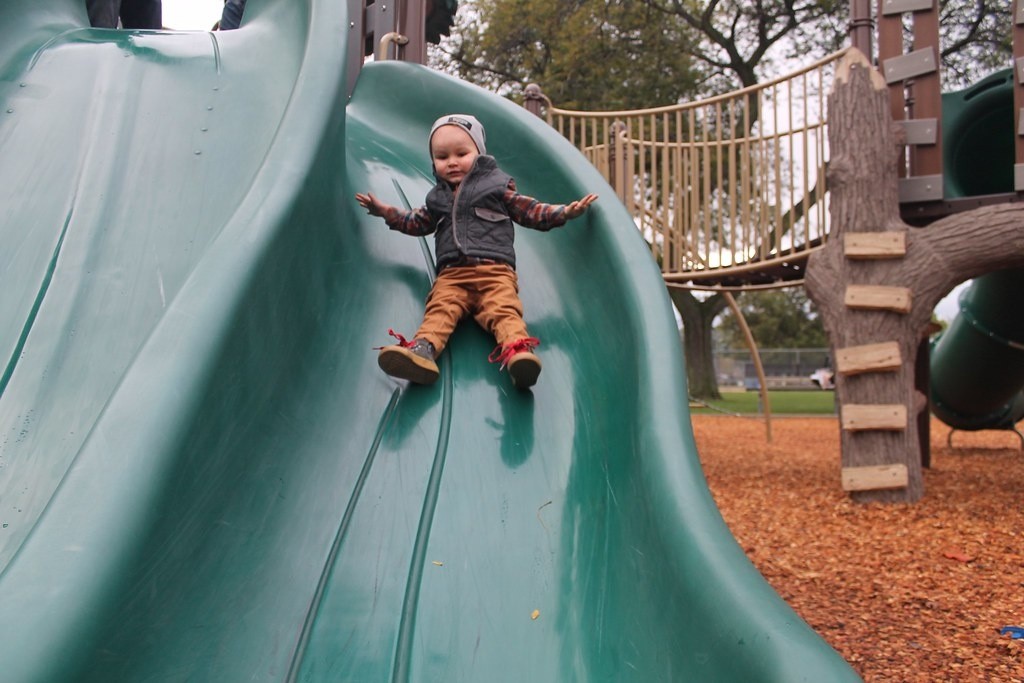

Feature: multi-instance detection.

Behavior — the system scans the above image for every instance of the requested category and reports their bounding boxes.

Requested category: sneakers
[373,330,440,387]
[488,336,542,388]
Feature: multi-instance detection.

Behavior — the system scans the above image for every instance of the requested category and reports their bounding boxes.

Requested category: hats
[430,115,486,158]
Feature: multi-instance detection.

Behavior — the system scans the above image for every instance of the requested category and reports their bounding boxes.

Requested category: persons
[355,114,600,387]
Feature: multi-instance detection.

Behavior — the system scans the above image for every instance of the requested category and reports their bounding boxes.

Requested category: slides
[0,0,864,683]
[927,66,1024,433]
